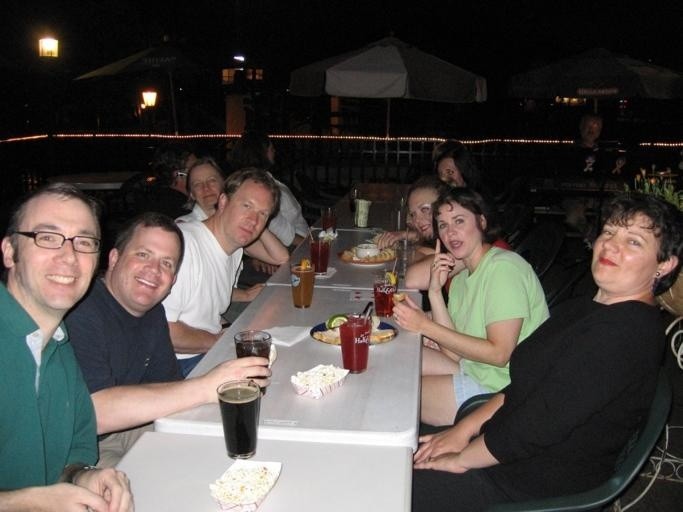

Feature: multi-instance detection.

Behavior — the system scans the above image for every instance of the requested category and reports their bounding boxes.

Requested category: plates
[308,321,398,346]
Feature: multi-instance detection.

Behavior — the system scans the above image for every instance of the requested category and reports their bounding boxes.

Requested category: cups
[288,262,314,309]
[348,189,359,212]
[320,207,337,234]
[339,313,372,374]
[373,282,397,318]
[233,330,271,394]
[215,378,260,459]
[308,237,330,275]
[380,268,398,294]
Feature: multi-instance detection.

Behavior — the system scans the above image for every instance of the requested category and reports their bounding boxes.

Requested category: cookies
[312,328,396,346]
[341,242,398,264]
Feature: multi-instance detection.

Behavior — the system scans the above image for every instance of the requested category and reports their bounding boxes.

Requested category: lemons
[386,271,396,287]
[327,313,348,330]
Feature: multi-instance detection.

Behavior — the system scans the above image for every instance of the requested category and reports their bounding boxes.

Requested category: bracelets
[61,463,102,485]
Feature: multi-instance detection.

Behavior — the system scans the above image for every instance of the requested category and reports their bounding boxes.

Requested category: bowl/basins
[354,242,380,258]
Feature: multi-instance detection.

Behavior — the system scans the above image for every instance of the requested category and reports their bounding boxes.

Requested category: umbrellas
[286,29,488,162]
[505,35,683,119]
[70,34,231,136]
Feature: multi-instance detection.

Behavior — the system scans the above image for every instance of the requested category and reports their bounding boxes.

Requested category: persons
[570,111,601,171]
[61,209,275,472]
[156,130,312,378]
[411,192,682,512]
[0,181,130,510]
[375,142,552,428]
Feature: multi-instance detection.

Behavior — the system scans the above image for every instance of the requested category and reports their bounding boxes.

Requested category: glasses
[16,232,101,253]
